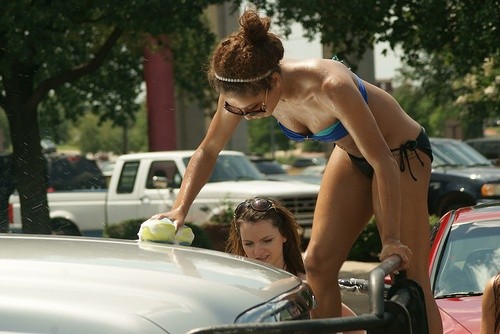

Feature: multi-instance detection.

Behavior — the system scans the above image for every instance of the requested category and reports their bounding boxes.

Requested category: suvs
[248,155,288,177]
[0,232,423,334]
[39,153,106,191]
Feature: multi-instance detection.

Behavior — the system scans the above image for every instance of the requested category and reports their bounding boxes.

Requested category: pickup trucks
[8,149,320,254]
[304,135,500,216]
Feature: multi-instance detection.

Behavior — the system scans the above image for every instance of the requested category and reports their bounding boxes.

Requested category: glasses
[223,88,268,115]
[233,198,278,234]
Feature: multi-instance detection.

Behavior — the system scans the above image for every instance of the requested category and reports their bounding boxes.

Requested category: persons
[150,8,444,334]
[221,197,367,333]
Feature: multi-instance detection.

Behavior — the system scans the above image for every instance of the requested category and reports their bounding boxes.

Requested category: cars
[425,203,500,334]
